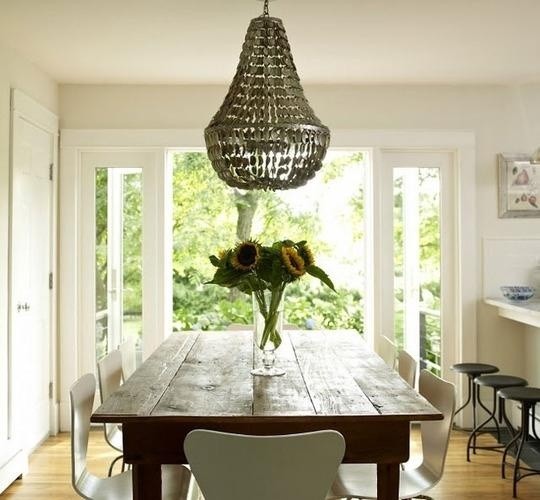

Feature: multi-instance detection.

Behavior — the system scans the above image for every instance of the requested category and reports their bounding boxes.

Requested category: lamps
[204,0,334,194]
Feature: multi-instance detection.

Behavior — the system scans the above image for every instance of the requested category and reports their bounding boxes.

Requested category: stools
[445,358,539,499]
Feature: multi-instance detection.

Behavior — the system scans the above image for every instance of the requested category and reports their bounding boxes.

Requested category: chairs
[70,373,195,499]
[178,425,347,500]
[96,350,161,479]
[329,333,457,500]
[116,334,142,385]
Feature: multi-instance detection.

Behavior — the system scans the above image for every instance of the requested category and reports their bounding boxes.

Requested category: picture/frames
[495,150,540,220]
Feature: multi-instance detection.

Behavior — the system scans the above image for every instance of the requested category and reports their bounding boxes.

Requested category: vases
[245,284,294,379]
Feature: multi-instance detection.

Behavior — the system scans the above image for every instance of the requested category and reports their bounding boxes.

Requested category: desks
[82,326,449,500]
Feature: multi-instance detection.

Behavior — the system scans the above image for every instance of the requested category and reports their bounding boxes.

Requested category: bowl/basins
[499,285,535,304]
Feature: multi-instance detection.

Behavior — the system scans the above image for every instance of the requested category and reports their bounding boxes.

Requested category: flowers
[196,234,339,358]
